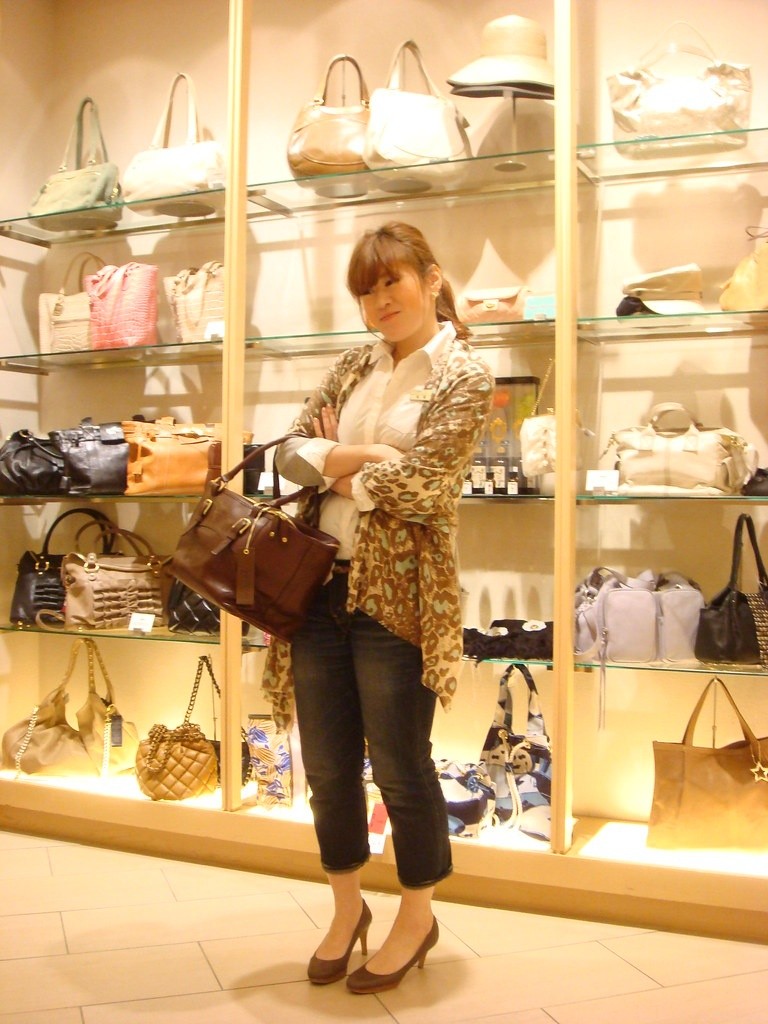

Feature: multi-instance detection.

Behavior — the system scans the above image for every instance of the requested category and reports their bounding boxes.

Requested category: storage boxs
[471,375,542,497]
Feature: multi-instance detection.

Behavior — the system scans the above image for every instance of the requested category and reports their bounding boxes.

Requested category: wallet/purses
[455,285,530,321]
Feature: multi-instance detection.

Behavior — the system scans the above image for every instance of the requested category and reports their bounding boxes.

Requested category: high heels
[307,897,374,983]
[345,916,440,995]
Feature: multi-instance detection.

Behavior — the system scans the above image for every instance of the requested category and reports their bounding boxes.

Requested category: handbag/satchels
[83,263,159,351]
[9,508,125,627]
[362,39,467,188]
[162,262,225,353]
[287,54,372,187]
[2,636,139,779]
[60,520,165,630]
[207,442,266,495]
[205,602,555,839]
[48,415,160,496]
[37,290,92,366]
[119,71,228,217]
[694,513,768,668]
[27,96,123,231]
[1,429,76,497]
[644,675,768,853]
[616,262,707,304]
[573,564,706,666]
[159,436,341,645]
[120,417,254,498]
[134,655,219,801]
[604,19,752,160]
[167,579,250,637]
[596,400,758,497]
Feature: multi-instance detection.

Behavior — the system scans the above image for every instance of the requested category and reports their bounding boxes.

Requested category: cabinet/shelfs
[225,0,564,917]
[559,0,768,944]
[0,0,237,864]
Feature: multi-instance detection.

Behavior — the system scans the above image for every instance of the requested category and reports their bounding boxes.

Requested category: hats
[444,14,554,99]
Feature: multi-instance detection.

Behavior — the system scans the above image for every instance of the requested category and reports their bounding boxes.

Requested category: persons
[275,222,496,993]
